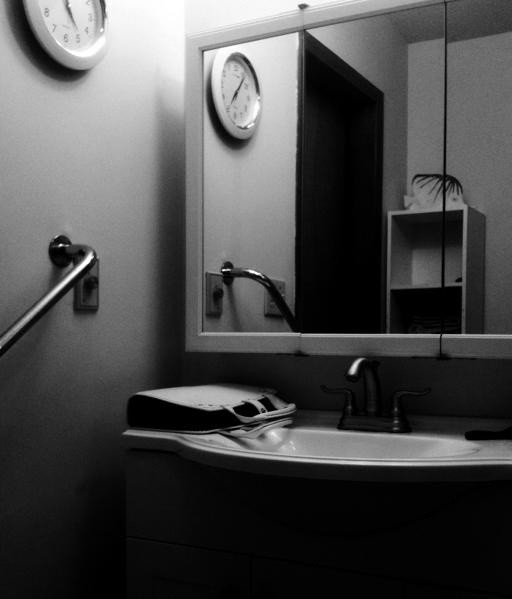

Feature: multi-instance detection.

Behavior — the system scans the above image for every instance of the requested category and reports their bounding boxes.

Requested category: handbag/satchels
[126,382,297,440]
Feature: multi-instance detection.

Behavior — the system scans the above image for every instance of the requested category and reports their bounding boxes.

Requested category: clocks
[19,0,111,71]
[209,47,263,142]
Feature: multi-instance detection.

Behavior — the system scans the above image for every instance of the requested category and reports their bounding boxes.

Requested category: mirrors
[182,0,512,360]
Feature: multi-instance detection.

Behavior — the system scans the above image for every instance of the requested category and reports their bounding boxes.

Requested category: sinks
[198,423,481,463]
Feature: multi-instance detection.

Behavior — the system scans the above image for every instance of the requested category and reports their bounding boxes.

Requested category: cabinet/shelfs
[386,208,486,334]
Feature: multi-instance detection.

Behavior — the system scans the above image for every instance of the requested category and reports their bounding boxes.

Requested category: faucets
[345,355,383,430]
